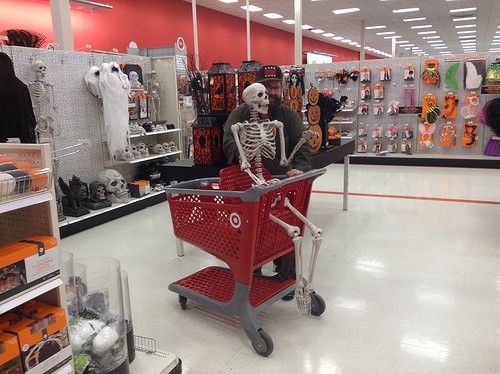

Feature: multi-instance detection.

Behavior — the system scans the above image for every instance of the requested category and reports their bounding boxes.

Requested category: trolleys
[165,167,326,357]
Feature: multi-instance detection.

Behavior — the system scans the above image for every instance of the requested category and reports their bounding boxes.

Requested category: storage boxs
[0,301,70,374]
[0,235,61,301]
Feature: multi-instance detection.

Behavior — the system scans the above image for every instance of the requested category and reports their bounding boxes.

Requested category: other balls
[0,156,48,198]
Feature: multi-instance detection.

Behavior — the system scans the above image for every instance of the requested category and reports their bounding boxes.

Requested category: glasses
[265,84,284,92]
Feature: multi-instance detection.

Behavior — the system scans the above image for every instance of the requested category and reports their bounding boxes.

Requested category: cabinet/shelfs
[0,142,73,374]
[0,45,182,237]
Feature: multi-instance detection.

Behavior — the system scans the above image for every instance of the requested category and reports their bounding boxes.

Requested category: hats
[255,64,283,83]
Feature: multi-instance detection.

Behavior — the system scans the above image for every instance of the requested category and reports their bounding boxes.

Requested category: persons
[223,64,311,300]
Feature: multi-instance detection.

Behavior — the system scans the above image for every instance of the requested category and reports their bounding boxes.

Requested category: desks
[160,139,352,257]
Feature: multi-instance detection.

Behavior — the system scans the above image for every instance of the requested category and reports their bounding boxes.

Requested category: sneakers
[281,290,295,300]
